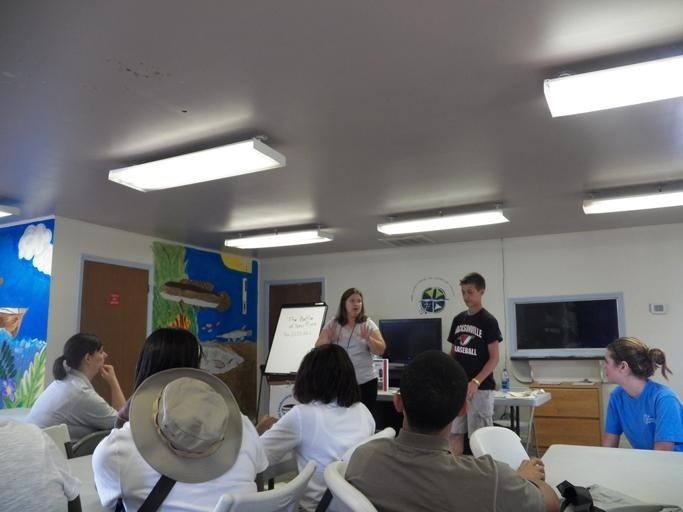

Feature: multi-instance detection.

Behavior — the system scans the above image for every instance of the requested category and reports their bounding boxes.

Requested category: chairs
[470,426,531,473]
[214,460,317,512]
[314,427,395,512]
[322,461,379,512]
[71,430,111,458]
[40,424,74,461]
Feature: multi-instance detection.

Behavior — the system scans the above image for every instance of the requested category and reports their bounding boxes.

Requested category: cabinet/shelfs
[529,381,602,459]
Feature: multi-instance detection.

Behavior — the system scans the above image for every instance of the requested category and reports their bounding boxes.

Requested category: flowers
[1,381,16,404]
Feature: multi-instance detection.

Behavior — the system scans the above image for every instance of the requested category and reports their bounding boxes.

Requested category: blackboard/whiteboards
[261,300,329,377]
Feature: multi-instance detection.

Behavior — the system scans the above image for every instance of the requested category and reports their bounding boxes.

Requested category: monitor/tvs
[507,291,626,360]
[378,317,443,371]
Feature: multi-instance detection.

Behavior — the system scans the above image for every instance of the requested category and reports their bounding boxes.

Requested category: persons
[23,333,126,441]
[91,328,268,512]
[258,344,376,512]
[0,417,82,512]
[447,272,503,457]
[601,336,683,451]
[345,351,561,511]
[315,288,386,414]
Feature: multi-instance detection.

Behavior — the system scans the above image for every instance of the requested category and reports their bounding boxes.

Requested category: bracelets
[472,378,481,386]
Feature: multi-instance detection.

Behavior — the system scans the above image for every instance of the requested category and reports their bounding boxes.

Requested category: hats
[128,367,242,484]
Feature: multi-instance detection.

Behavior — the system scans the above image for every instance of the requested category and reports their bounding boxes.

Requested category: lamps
[377,205,512,237]
[543,46,683,118]
[225,225,334,249]
[581,181,683,215]
[107,138,287,193]
[0,204,19,219]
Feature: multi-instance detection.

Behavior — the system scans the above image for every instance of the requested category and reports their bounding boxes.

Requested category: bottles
[501,368,510,394]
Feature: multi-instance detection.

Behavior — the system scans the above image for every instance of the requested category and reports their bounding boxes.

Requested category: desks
[376,388,551,454]
[535,444,683,512]
